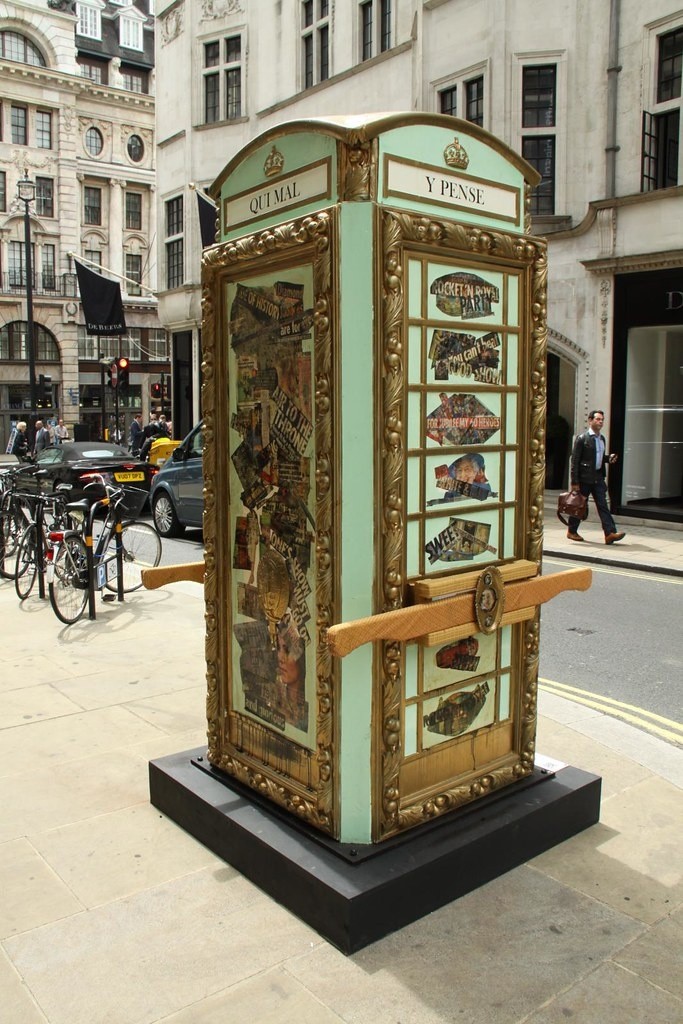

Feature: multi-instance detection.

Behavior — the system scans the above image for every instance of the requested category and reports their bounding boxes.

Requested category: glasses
[151,419,156,420]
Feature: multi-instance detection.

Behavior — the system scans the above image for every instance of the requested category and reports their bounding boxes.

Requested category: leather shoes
[605,532,625,544]
[567,531,583,541]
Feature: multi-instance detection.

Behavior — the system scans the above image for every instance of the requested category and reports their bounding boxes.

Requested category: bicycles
[0,465,162,625]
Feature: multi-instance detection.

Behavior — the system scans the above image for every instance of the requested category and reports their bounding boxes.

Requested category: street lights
[16,167,37,449]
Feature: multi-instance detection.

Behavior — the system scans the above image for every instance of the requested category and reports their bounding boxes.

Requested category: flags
[73,258,127,336]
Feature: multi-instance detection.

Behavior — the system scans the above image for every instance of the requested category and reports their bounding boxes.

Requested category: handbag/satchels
[557,490,588,527]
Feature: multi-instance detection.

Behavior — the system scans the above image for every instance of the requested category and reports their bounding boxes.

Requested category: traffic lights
[39,374,52,400]
[107,357,130,390]
[151,383,161,398]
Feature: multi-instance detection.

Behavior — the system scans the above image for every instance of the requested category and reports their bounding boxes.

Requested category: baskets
[109,483,150,520]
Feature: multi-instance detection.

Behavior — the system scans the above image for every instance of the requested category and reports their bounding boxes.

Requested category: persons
[142,414,172,443]
[567,410,625,545]
[130,414,144,455]
[276,634,306,711]
[46,420,68,445]
[444,457,490,498]
[32,421,51,456]
[12,421,28,464]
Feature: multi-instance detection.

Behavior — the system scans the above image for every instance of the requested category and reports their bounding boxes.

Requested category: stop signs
[112,364,117,387]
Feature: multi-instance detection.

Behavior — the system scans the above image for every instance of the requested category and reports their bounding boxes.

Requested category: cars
[148,419,203,538]
[8,442,160,512]
